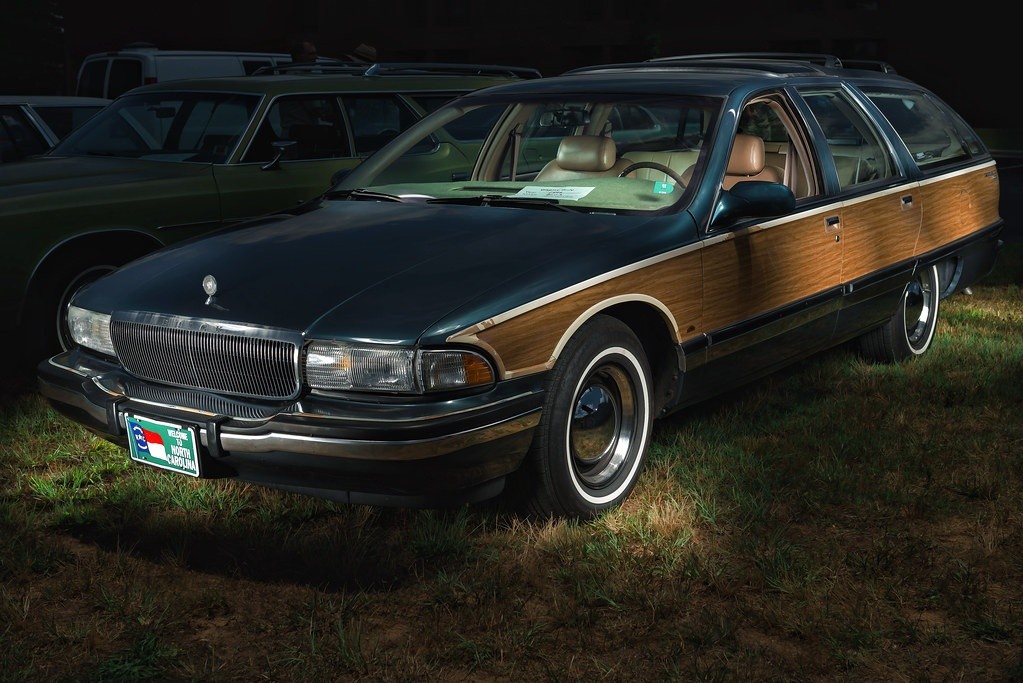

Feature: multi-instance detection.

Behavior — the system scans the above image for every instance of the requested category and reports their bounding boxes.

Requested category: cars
[0,47,699,377]
[38,54,1006,521]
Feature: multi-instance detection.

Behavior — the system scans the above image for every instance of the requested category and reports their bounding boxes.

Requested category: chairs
[287,124,339,160]
[533,135,637,181]
[676,133,779,191]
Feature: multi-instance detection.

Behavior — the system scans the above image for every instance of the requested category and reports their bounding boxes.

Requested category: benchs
[622,151,874,187]
[829,143,950,165]
